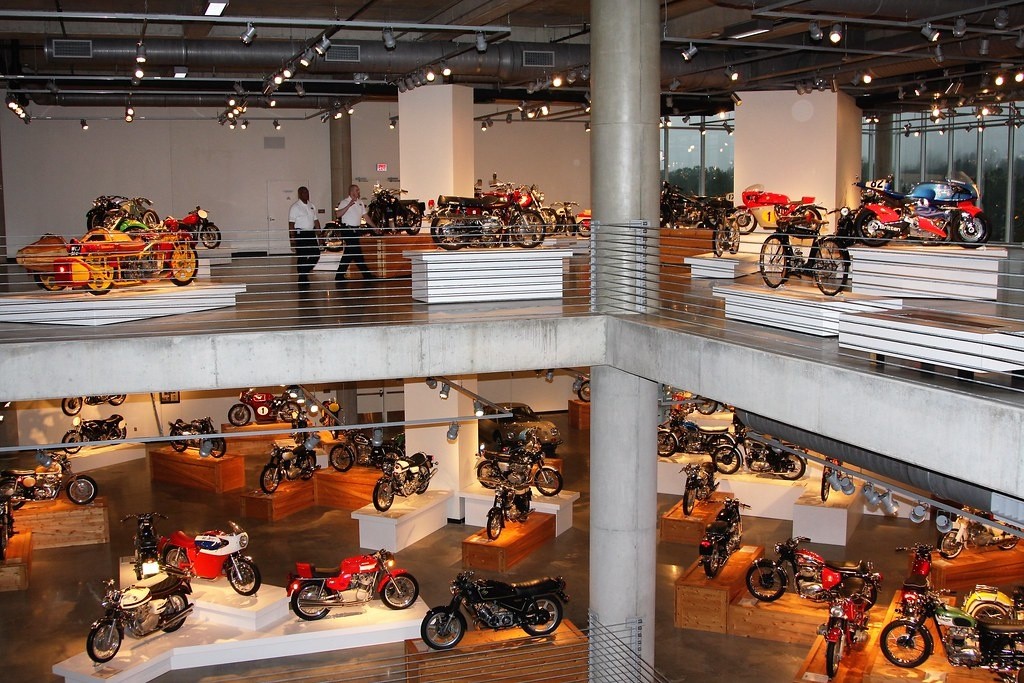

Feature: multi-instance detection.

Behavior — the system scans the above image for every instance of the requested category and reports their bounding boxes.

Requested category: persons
[288,187,326,289]
[474,179,481,189]
[335,185,382,283]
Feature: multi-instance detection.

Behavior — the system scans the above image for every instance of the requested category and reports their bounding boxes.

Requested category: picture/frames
[160,392,180,403]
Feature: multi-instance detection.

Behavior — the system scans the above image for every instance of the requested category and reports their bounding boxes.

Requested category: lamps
[480,66,591,132]
[866,112,882,123]
[427,378,483,439]
[35,449,52,467]
[218,21,355,130]
[826,468,953,534]
[536,368,554,381]
[572,373,584,391]
[475,30,487,50]
[398,60,452,93]
[772,437,783,453]
[126,43,147,122]
[723,65,739,81]
[660,78,742,135]
[794,13,873,94]
[681,41,697,61]
[80,119,88,130]
[199,440,213,457]
[897,11,1024,138]
[5,94,32,124]
[382,25,396,48]
[47,79,58,94]
[287,387,320,448]
[390,120,397,130]
[373,427,384,446]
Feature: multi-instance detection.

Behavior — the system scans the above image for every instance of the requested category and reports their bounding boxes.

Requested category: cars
[478,401,564,454]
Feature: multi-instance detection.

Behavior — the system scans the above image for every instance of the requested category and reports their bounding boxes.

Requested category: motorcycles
[259,432,322,495]
[474,425,564,540]
[820,455,843,502]
[937,510,1021,560]
[0,448,98,511]
[372,448,439,512]
[14,194,223,297]
[329,428,405,476]
[119,511,169,580]
[168,415,227,458]
[421,569,571,650]
[679,461,720,516]
[61,413,127,454]
[659,169,993,258]
[86,573,194,664]
[879,583,1024,683]
[0,493,20,561]
[813,576,873,681]
[286,548,420,622]
[744,535,886,612]
[657,382,810,518]
[156,520,262,597]
[894,541,942,633]
[697,495,752,579]
[61,393,127,416]
[227,384,307,427]
[319,397,340,440]
[317,171,591,253]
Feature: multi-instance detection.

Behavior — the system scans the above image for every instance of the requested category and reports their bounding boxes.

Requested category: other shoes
[363,276,373,279]
[335,275,349,283]
[298,278,311,285]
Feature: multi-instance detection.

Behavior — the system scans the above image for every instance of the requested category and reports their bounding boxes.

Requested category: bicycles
[758,217,851,297]
